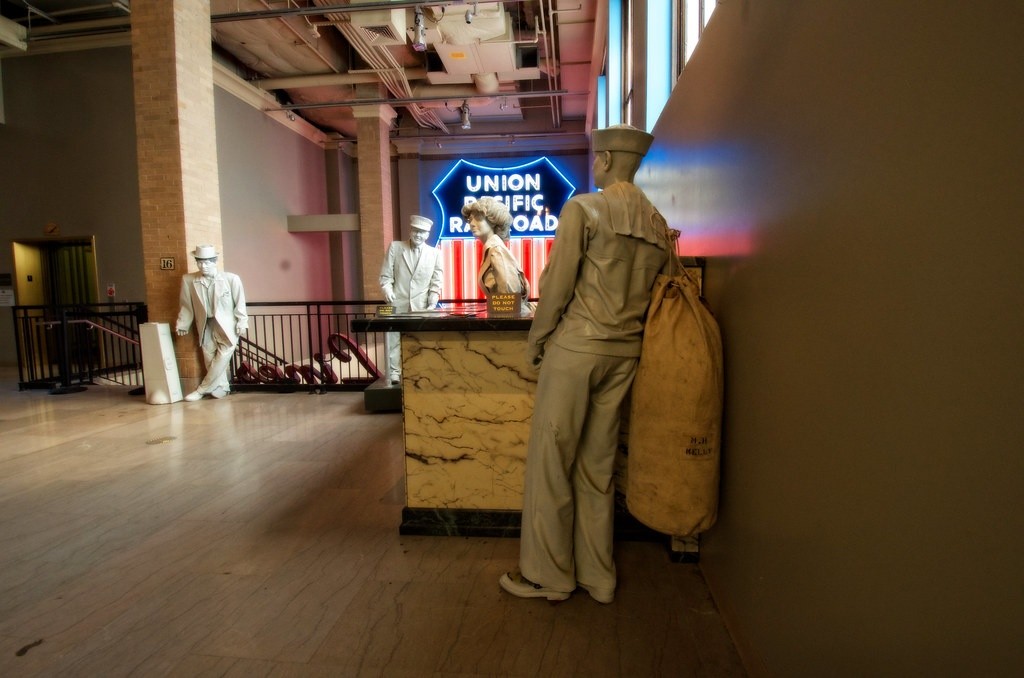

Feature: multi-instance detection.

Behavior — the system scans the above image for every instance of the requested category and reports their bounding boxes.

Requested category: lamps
[412,10,428,52]
[460,106,471,129]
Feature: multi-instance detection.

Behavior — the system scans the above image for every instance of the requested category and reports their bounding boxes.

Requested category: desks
[351,291,709,537]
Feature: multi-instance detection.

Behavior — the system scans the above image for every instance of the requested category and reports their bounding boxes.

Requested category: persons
[379,215,443,383]
[176,245,248,401]
[462,199,533,317]
[500,127,672,603]
